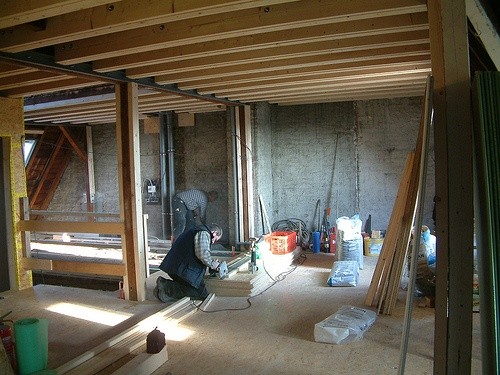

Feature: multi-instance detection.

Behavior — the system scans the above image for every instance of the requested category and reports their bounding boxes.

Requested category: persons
[172,190,218,238]
[156,223,223,303]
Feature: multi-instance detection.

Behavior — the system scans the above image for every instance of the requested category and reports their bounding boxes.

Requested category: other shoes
[156,277,169,302]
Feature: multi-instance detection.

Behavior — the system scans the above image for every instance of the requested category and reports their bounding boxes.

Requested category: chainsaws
[216,261,227,281]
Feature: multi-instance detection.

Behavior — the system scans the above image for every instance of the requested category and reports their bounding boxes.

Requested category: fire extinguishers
[1,308,16,369]
